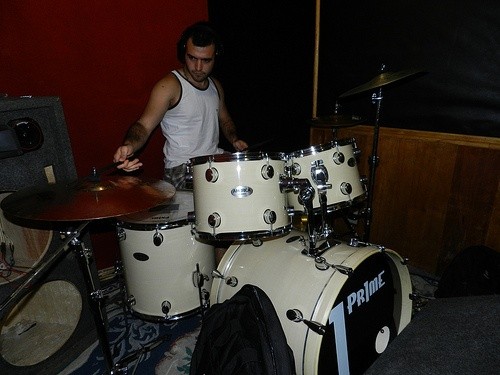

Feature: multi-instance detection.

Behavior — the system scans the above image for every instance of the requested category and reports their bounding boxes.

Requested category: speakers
[0,94,78,195]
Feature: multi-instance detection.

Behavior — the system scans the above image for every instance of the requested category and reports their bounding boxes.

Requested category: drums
[190,149,298,247]
[115,188,215,320]
[286,138,364,220]
[210,228,414,374]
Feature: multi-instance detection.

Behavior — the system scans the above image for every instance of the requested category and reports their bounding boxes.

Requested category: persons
[114,20,249,269]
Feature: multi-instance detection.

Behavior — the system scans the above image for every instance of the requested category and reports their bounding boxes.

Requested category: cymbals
[308,117,367,129]
[339,70,423,98]
[4,174,176,223]
